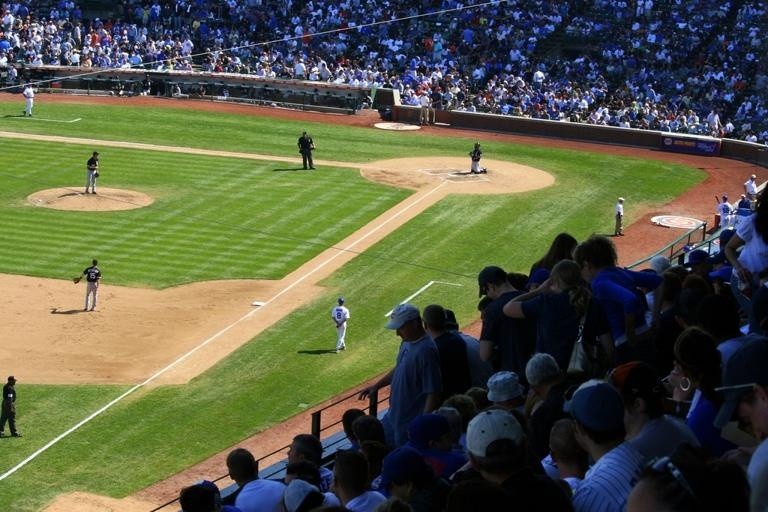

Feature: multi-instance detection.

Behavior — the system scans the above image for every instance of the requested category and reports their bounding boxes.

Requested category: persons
[86,152,99,194]
[74,260,101,311]
[181,176,767,511]
[298,132,315,169]
[0,0,768,145]
[0,376,19,436]
[470,135,486,174]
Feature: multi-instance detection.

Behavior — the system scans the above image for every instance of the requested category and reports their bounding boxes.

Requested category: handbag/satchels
[567,326,616,376]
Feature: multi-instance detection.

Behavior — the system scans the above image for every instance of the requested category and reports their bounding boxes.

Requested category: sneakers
[342,344,346,351]
[336,346,340,353]
[92,191,97,194]
[0,431,5,437]
[84,307,88,310]
[12,432,22,437]
[91,307,95,310]
[85,190,90,194]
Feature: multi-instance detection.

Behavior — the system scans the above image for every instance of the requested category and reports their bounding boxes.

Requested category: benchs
[670,225,736,272]
[219,408,391,505]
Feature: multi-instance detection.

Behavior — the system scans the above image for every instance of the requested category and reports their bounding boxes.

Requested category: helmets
[338,298,345,305]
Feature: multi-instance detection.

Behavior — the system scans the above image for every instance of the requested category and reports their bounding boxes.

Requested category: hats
[617,196,625,202]
[525,354,561,382]
[525,268,550,291]
[608,360,655,394]
[487,370,525,404]
[651,255,671,272]
[401,412,450,448]
[465,409,525,460]
[93,152,100,155]
[477,266,507,298]
[8,376,18,383]
[683,249,710,268]
[570,379,628,440]
[712,333,768,428]
[370,445,425,490]
[708,265,733,281]
[384,304,421,333]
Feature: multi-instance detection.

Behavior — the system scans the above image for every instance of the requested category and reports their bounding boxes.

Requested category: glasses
[648,454,698,502]
